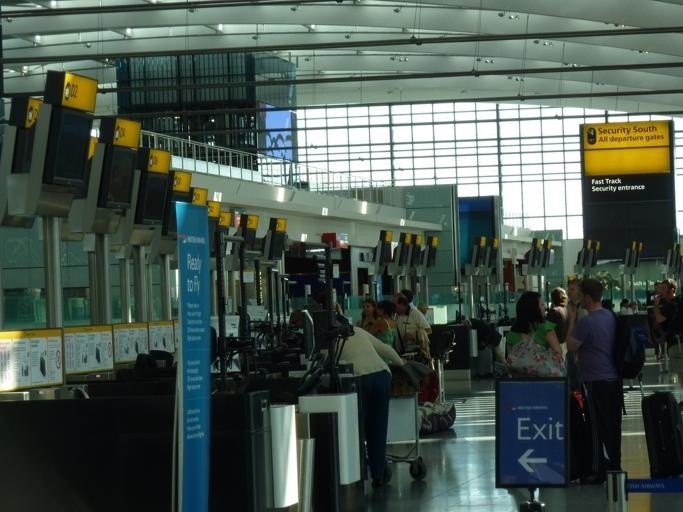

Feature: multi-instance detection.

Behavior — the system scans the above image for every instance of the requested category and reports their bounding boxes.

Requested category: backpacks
[614,319,646,379]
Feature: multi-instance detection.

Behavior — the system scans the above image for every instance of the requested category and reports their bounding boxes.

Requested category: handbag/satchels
[506,339,568,378]
[413,400,456,434]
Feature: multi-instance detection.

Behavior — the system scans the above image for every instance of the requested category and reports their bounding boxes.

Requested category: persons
[332,288,432,397]
[505,273,682,414]
[565,277,623,485]
[333,313,410,488]
[466,317,509,377]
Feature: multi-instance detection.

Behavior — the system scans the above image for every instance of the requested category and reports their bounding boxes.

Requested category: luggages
[640,390,683,479]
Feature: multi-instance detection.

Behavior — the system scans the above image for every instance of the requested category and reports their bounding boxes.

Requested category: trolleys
[358,360,427,482]
